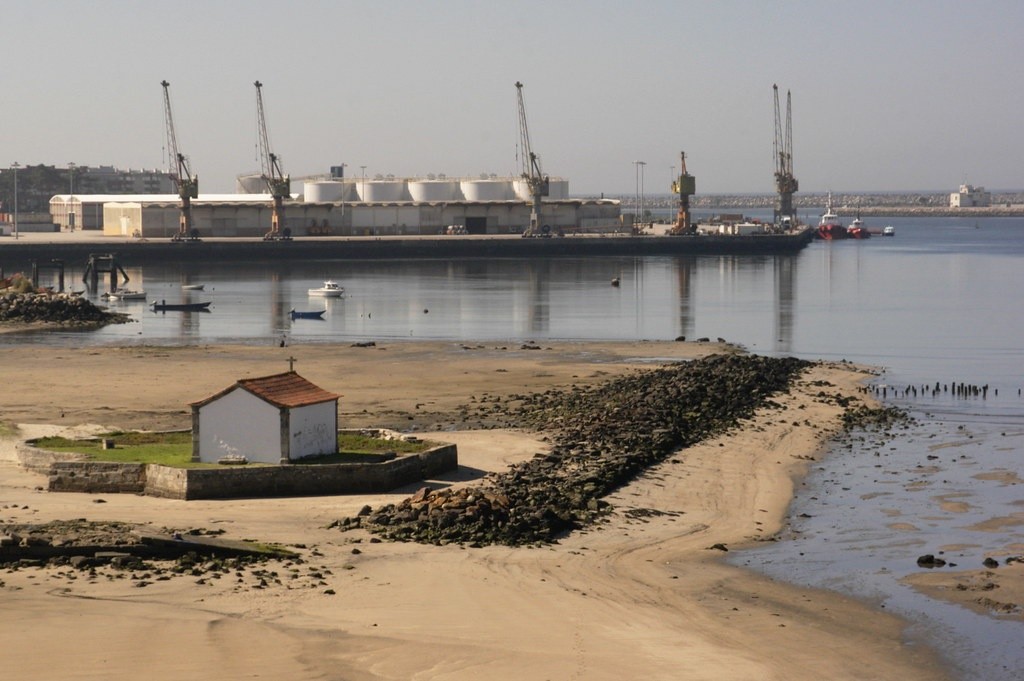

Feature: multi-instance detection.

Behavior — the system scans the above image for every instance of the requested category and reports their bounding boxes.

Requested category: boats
[288,309,327,319]
[881,224,895,236]
[308,279,345,297]
[104,288,147,299]
[845,204,871,240]
[181,284,205,290]
[815,190,847,242]
[154,299,212,311]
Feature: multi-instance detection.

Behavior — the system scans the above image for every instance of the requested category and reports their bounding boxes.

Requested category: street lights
[361,165,367,202]
[341,163,348,216]
[68,161,76,226]
[669,166,676,224]
[639,161,647,222]
[11,162,21,240]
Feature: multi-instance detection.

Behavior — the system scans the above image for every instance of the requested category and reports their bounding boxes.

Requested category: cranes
[773,83,799,228]
[253,80,294,241]
[160,80,204,243]
[669,150,699,236]
[513,80,551,238]
